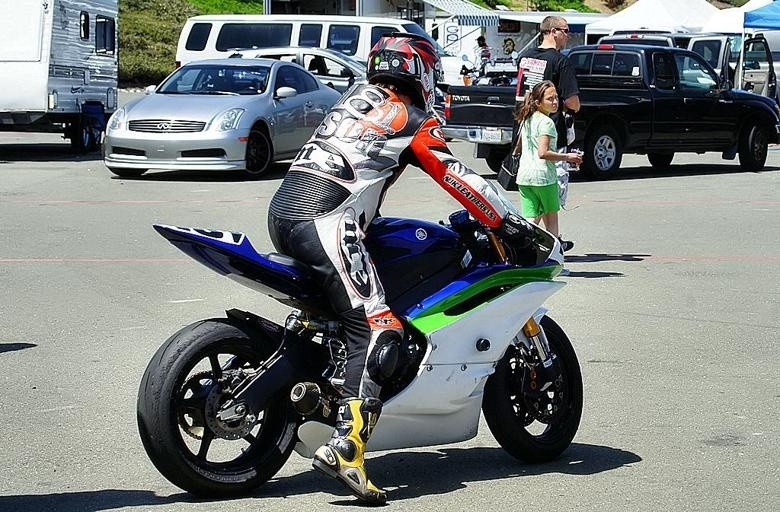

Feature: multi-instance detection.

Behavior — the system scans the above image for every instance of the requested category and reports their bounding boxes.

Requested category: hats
[475,36,485,41]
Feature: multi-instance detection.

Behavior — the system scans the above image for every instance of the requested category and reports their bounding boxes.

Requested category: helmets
[368,32,440,114]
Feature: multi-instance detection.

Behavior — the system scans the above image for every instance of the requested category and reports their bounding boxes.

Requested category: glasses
[555,28,568,34]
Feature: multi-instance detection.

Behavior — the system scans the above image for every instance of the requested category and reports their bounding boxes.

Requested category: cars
[101,57,343,183]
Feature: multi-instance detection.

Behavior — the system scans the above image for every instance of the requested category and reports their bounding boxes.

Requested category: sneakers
[558,237,573,252]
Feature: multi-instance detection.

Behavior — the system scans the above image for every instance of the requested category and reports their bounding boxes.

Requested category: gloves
[493,214,545,248]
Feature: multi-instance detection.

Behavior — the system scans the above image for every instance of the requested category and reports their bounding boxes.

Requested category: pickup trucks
[436,39,779,184]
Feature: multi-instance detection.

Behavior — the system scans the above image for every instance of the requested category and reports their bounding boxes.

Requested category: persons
[474,35,491,62]
[512,16,581,205]
[503,39,516,55]
[512,81,582,254]
[267,32,509,506]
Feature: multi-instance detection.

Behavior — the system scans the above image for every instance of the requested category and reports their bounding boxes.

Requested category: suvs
[216,43,447,131]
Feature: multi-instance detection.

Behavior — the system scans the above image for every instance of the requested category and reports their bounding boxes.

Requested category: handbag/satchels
[563,111,576,145]
[498,155,519,191]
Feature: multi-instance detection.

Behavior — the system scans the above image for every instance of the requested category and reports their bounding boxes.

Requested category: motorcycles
[133,189,582,498]
[459,47,515,89]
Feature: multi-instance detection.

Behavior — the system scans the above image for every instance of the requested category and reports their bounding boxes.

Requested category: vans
[170,10,480,113]
[494,24,779,107]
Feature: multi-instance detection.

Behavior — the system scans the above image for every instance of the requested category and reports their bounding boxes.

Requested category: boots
[312,397,387,503]
